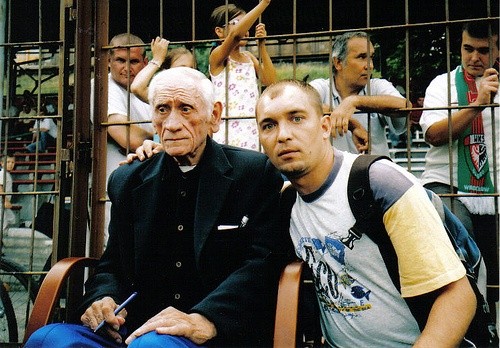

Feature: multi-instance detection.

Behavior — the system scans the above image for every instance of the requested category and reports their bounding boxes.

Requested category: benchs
[384,125,433,172]
[0,133,77,182]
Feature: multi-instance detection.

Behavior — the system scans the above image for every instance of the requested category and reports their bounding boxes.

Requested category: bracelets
[148,58,162,68]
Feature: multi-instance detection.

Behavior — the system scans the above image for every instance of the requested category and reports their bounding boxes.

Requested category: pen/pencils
[94,291,137,332]
[239,215,248,227]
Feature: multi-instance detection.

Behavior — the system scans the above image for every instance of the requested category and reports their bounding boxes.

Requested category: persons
[128,35,198,105]
[90,31,163,254]
[119,77,480,348]
[381,70,430,149]
[419,20,500,323]
[207,0,281,154]
[307,31,412,161]
[24,67,283,348]
[0,94,60,229]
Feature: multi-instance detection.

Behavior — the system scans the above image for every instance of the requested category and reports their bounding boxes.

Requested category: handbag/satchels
[29,194,55,239]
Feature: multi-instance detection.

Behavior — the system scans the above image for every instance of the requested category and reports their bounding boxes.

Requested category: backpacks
[277,155,496,348]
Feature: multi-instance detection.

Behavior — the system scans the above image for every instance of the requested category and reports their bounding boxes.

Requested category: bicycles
[0,279,19,344]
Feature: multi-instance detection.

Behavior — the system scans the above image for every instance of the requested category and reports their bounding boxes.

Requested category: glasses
[222,18,239,26]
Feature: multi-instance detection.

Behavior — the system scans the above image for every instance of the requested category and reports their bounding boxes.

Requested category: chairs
[23,256,304,348]
